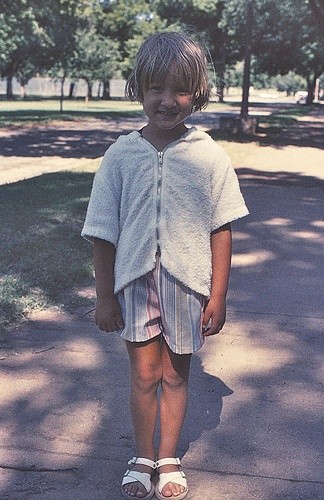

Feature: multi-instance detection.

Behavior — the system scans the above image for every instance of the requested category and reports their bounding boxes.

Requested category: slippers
[121,457,157,500]
[153,458,189,500]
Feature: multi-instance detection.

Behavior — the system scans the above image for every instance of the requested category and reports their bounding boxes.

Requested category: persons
[80,30,251,500]
